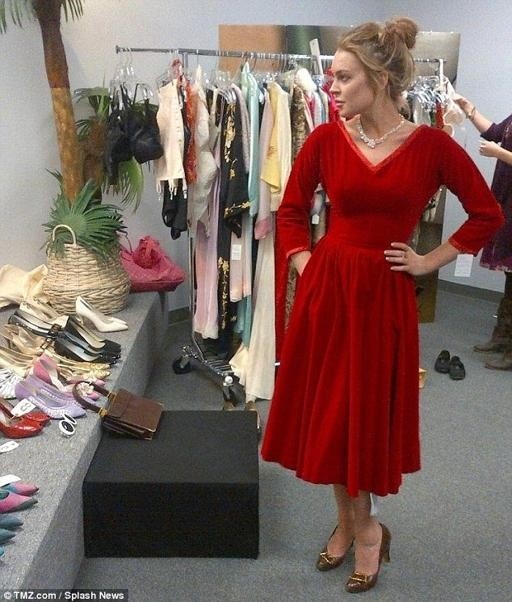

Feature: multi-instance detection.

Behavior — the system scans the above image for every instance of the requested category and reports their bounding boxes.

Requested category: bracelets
[466,105,476,121]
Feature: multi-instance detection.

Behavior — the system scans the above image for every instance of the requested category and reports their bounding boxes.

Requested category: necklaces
[356,113,404,148]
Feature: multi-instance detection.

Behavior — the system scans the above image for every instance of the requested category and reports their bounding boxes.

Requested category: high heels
[344,521,391,593]
[315,523,357,571]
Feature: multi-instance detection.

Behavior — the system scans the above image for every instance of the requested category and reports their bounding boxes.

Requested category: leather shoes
[0,294,128,559]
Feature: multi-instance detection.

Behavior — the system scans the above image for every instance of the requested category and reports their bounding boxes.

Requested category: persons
[445,76,512,371]
[259,17,506,594]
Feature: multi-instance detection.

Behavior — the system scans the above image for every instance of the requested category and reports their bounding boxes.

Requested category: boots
[487,352,512,370]
[474,297,511,353]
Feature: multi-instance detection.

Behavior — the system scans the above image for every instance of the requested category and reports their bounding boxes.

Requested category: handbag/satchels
[74,381,166,440]
[118,234,187,292]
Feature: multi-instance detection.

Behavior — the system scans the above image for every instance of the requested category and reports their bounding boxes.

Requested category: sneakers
[449,356,465,379]
[435,350,450,372]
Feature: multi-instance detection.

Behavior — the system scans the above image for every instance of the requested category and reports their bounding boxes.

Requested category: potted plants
[39,166,131,316]
